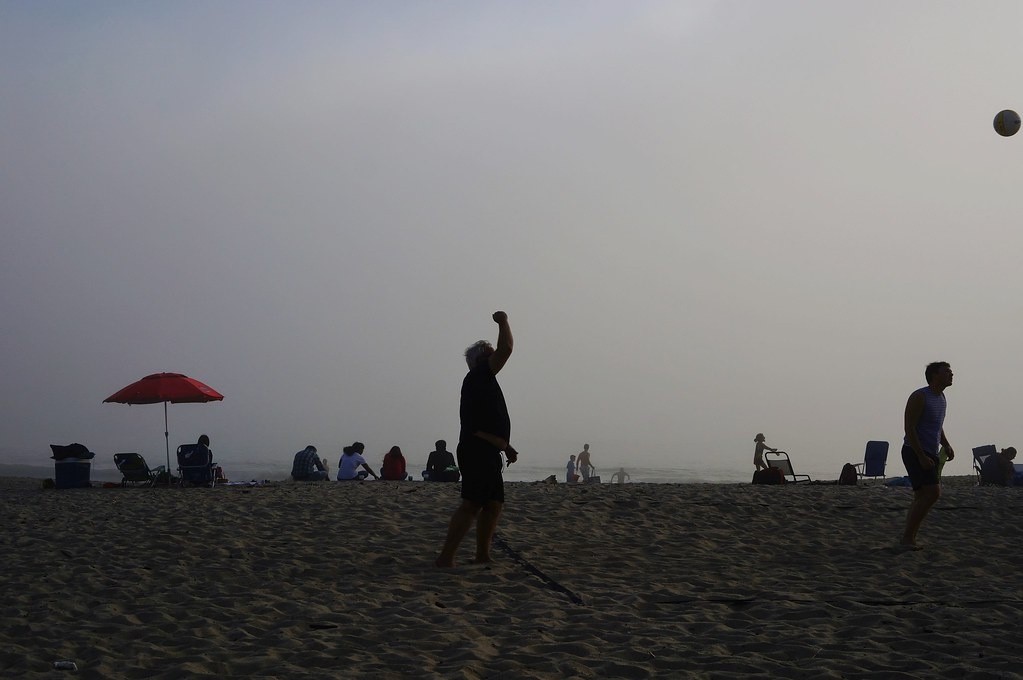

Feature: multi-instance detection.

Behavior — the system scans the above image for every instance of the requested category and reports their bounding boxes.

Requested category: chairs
[113,452,165,488]
[765,451,812,485]
[972,445,995,486]
[177,443,219,489]
[854,441,889,488]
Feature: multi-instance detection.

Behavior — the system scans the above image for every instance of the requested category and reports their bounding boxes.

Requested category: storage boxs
[54,458,91,489]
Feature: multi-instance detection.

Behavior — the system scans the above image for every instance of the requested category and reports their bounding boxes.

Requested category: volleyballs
[993,110,1022,137]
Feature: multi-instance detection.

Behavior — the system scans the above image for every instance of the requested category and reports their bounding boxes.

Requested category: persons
[337,442,379,480]
[189,434,213,464]
[380,446,408,480]
[567,455,576,481]
[422,440,456,480]
[754,433,780,470]
[901,362,954,550]
[985,447,1016,481]
[611,468,629,483]
[291,445,328,480]
[576,444,594,482]
[436,311,517,566]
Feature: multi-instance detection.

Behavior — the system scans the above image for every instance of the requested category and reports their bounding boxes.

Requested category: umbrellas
[102,371,223,486]
